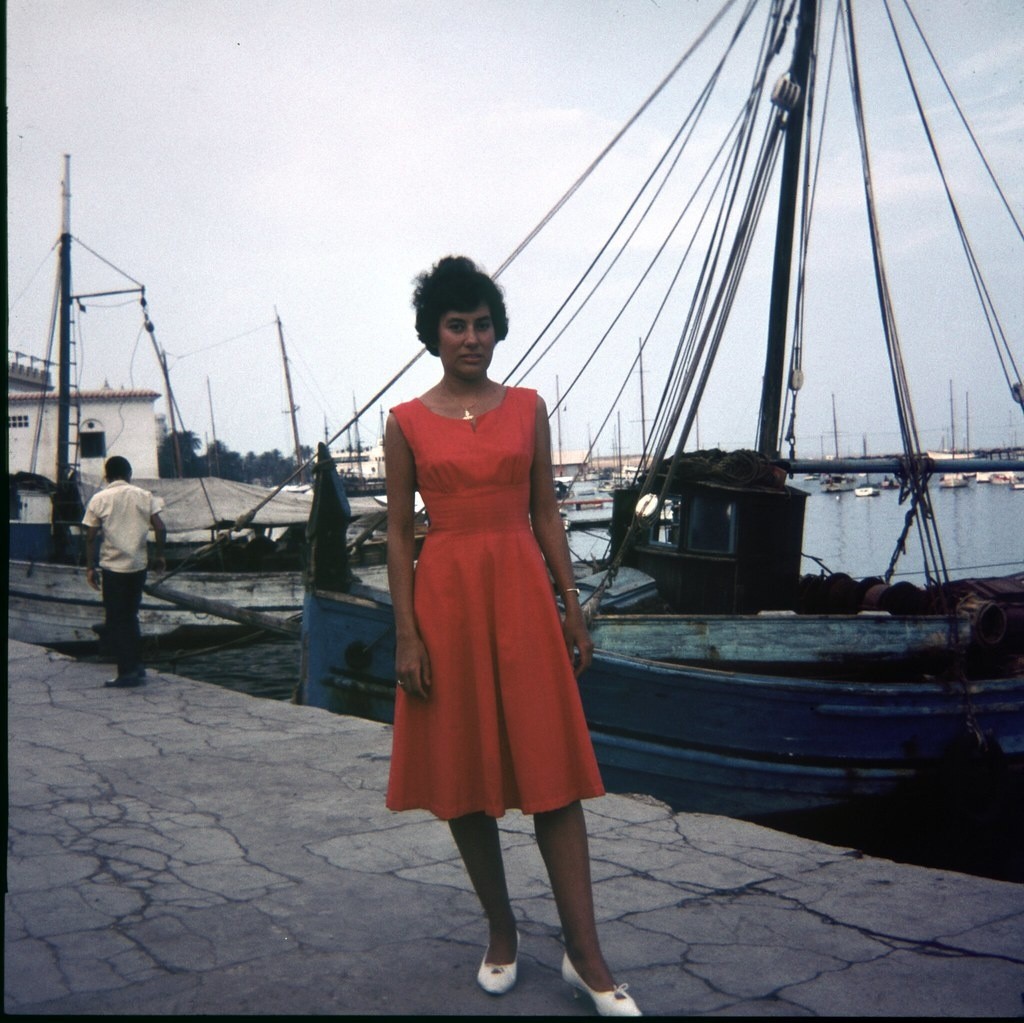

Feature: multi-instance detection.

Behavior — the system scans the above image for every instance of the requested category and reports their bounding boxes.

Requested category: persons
[385,256,644,1018]
[82,456,168,689]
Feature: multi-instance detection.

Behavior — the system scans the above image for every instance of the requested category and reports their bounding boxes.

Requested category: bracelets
[560,587,581,596]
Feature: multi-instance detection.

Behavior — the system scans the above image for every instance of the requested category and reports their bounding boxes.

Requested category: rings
[397,681,404,684]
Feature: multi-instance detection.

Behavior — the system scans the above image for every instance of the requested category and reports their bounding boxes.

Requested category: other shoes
[105,671,149,686]
[477,930,521,995]
[562,953,642,1016]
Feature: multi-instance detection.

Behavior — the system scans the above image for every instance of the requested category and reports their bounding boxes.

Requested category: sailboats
[9,141,431,656]
[374,378,1024,536]
[296,0,1024,841]
